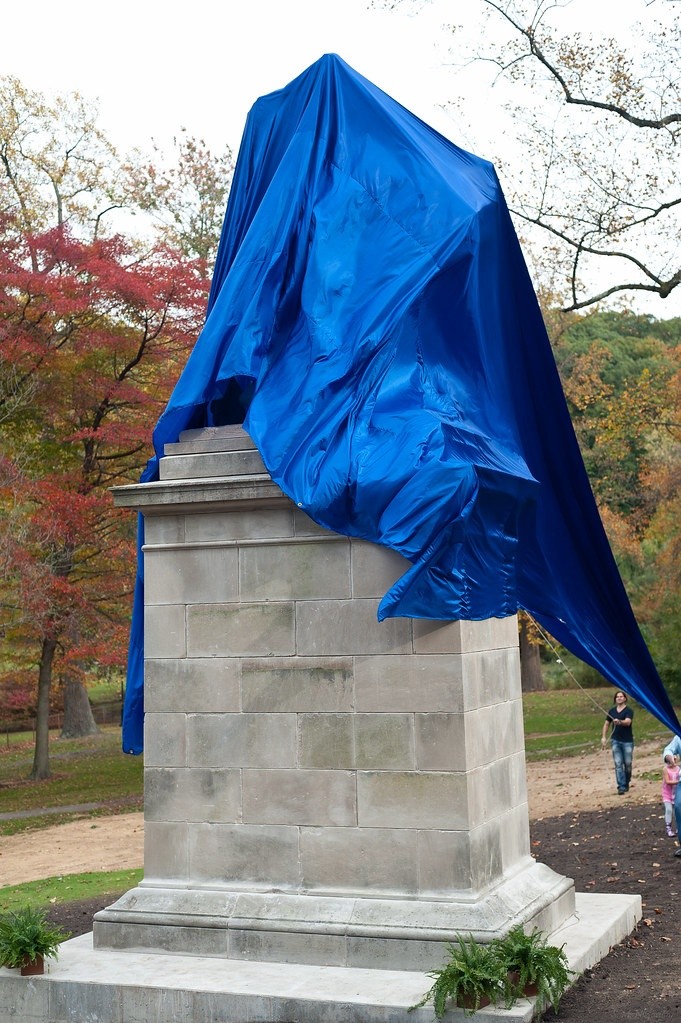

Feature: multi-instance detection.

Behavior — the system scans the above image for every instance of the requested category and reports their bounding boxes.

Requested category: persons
[600,691,634,795]
[662,735,681,855]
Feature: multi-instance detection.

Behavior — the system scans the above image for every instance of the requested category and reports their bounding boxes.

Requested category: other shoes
[666,827,676,837]
[618,789,624,795]
[624,786,630,791]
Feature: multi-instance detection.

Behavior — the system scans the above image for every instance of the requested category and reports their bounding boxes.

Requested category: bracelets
[618,720,621,726]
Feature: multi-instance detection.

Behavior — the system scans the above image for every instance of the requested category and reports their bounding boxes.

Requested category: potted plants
[407,924,582,1020]
[0,903,73,976]
